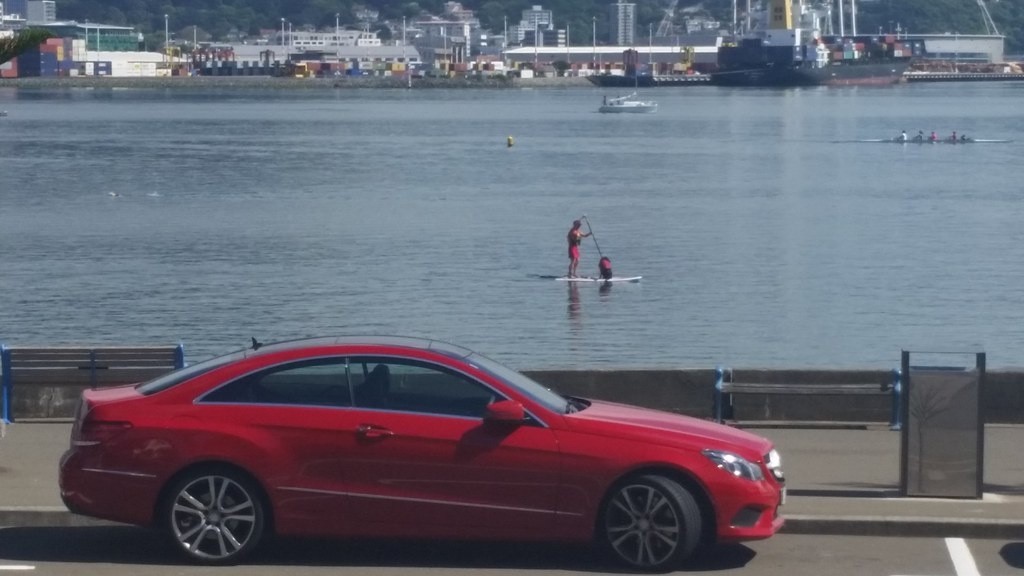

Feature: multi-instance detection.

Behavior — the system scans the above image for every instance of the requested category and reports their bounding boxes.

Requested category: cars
[57,334,790,575]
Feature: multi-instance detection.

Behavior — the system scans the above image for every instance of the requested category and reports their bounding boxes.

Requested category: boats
[598,93,657,111]
[586,0,916,90]
[837,137,1017,143]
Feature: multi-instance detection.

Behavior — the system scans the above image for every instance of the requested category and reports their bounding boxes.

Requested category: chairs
[236,372,272,402]
[365,364,400,409]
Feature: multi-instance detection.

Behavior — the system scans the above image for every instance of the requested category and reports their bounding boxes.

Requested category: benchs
[1,343,189,424]
[714,365,903,432]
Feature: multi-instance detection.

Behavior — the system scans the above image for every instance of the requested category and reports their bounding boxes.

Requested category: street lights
[280,17,285,55]
[335,12,341,60]
[503,15,508,47]
[288,22,292,50]
[591,16,596,68]
[85,18,89,50]
[163,14,170,54]
[649,22,653,64]
[402,16,406,59]
[193,24,197,52]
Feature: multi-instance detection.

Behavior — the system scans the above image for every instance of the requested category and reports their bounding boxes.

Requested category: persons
[897,130,957,141]
[566,216,592,279]
